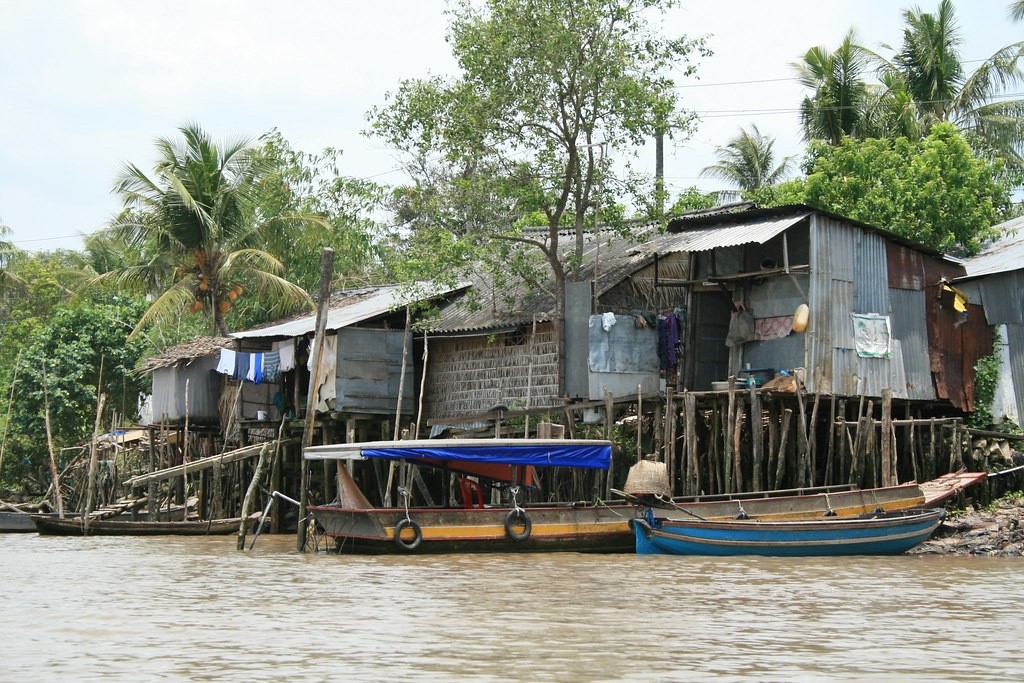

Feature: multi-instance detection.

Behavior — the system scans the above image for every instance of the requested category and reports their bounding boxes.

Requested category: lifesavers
[505,508,531,542]
[393,518,422,551]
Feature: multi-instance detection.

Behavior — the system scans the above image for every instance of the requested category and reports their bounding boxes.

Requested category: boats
[31,510,263,538]
[301,435,988,558]
[611,474,948,558]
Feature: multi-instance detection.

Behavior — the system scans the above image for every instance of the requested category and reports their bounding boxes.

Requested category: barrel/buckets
[257,411,267,420]
[792,303,810,332]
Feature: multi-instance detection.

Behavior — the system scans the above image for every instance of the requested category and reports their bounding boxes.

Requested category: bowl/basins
[711,381,746,391]
